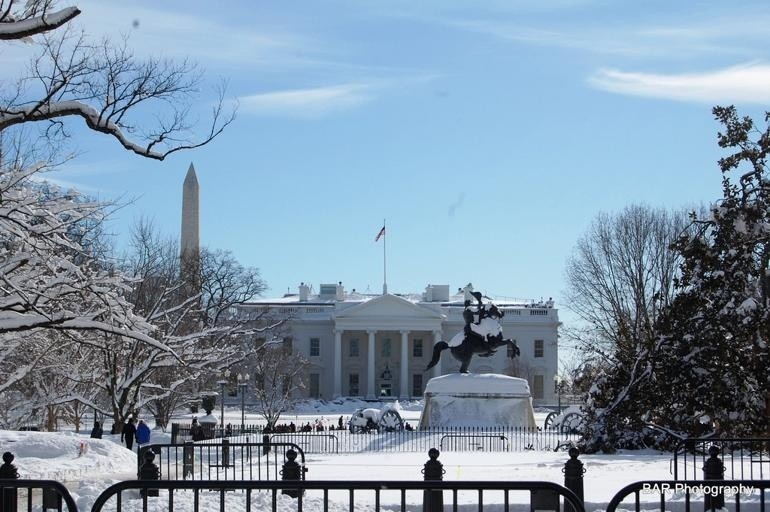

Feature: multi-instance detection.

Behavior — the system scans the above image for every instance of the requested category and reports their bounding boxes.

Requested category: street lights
[216,369,230,439]
[553,375,566,414]
[237,372,249,430]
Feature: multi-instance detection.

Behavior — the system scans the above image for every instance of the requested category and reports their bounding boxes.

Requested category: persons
[462,298,486,345]
[121,417,138,450]
[189,417,206,441]
[225,422,232,437]
[135,420,151,447]
[338,415,344,429]
[304,421,312,431]
[90,421,104,439]
[289,421,296,432]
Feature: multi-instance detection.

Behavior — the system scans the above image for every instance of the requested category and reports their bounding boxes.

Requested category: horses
[425,301,522,374]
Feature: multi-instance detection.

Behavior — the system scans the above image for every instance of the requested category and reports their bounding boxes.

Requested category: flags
[375,227,385,241]
